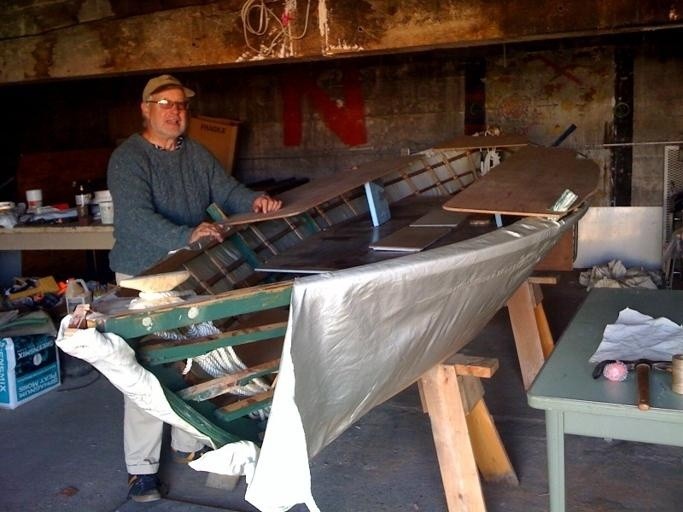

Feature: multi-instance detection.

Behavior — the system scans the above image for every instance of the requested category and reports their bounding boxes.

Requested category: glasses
[145,99,190,111]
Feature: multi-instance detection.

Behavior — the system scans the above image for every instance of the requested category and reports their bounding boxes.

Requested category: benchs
[147,350,520,512]
[499,270,560,391]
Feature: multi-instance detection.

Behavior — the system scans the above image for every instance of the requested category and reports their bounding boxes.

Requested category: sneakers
[127,473,162,502]
[171,445,212,463]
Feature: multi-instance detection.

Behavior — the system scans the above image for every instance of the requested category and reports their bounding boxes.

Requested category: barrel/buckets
[65,278,94,311]
[95,189,114,225]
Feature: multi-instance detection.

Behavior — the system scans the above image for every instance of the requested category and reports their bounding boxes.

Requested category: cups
[26,190,42,209]
[99,201,114,224]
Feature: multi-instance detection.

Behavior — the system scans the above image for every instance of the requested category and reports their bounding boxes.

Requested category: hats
[143,74,196,100]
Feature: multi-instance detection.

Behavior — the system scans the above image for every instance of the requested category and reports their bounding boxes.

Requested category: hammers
[622,359,672,411]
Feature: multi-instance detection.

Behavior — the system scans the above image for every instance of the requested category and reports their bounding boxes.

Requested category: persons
[106,73,282,501]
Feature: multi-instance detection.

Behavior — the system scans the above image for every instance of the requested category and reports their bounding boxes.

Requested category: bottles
[75,179,92,213]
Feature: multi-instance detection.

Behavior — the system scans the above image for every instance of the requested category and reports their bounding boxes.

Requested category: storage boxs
[0,335,62,411]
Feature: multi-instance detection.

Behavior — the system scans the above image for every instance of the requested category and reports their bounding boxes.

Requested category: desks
[527,287,683,512]
[0,218,116,250]
